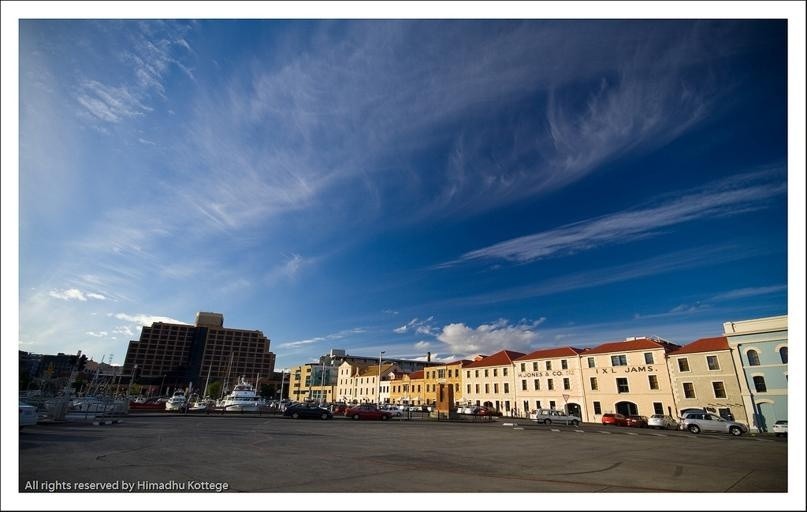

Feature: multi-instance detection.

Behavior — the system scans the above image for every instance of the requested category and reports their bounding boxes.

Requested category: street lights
[377,351,385,410]
[279,370,289,409]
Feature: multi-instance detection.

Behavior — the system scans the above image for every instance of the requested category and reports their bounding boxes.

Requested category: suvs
[679,409,748,437]
[529,408,580,426]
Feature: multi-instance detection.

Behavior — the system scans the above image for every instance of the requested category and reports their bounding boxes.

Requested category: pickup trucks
[772,420,788,437]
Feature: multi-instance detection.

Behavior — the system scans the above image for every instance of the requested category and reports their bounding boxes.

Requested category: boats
[44,352,220,413]
[219,379,265,411]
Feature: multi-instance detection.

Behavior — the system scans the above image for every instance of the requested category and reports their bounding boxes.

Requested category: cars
[602,412,679,431]
[455,405,503,417]
[18,401,39,430]
[270,398,433,420]
